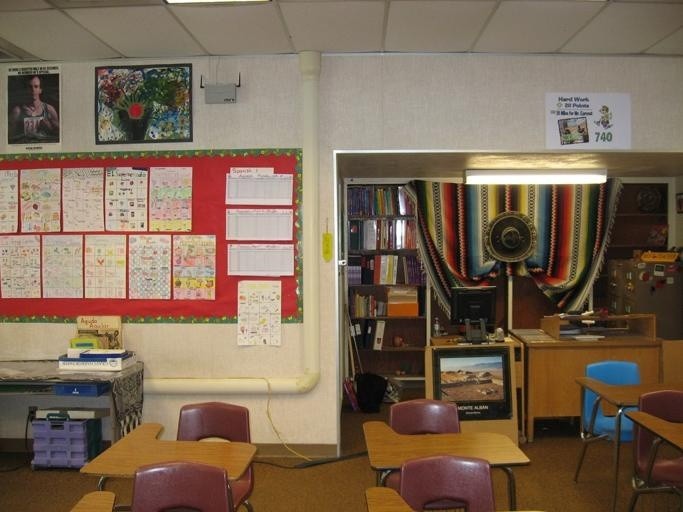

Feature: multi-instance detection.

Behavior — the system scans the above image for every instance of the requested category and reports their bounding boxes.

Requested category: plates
[636,187,661,211]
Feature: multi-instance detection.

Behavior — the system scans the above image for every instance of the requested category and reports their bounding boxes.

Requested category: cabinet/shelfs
[604,257,682,341]
[341,175,431,408]
[505,176,678,341]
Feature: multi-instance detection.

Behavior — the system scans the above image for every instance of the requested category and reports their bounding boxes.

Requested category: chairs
[175,401,254,512]
[379,399,461,486]
[574,361,682,512]
[131,459,233,511]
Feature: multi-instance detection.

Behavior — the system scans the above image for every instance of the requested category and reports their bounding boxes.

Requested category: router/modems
[200,72,241,104]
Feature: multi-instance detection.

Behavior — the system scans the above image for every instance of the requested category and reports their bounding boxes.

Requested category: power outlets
[25,405,37,422]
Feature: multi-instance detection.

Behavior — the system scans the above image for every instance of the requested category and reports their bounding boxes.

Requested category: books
[560,325,605,342]
[348,187,426,318]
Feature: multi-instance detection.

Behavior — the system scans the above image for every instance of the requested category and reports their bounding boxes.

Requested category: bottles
[431,317,440,337]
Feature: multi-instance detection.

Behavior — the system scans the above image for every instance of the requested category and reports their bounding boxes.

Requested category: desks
[70,492,116,511]
[427,333,525,446]
[506,324,680,444]
[362,420,532,511]
[79,421,259,492]
[0,359,147,449]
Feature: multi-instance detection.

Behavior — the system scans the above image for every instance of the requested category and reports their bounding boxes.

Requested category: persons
[8,75,59,144]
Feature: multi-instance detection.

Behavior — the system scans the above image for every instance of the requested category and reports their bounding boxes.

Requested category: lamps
[461,168,608,187]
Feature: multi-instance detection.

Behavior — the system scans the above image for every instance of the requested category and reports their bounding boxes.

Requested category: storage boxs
[28,419,102,471]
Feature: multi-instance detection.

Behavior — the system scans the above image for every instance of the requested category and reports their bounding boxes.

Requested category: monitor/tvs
[450,286,498,345]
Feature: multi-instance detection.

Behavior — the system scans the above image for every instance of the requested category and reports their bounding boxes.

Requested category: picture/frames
[91,63,194,146]
